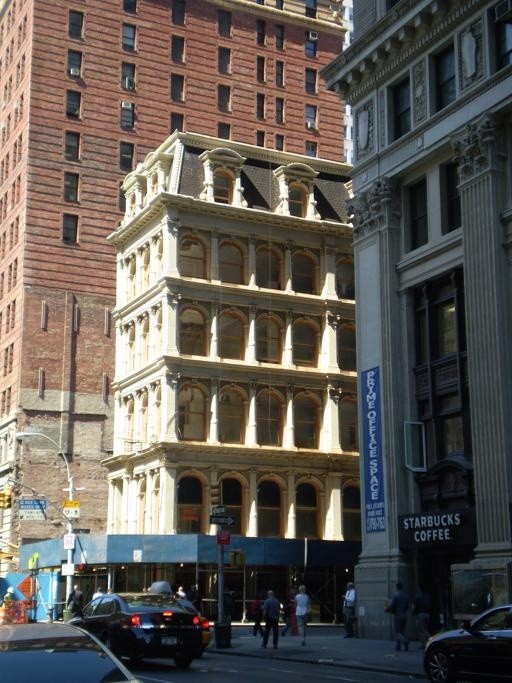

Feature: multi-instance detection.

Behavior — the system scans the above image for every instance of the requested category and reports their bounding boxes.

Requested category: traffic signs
[207,505,235,545]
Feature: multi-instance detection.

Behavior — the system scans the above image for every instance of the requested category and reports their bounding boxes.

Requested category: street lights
[16,432,75,621]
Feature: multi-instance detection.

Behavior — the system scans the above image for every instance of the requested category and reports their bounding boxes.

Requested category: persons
[341,581,358,639]
[90,587,104,613]
[383,581,411,653]
[141,581,312,651]
[1,586,17,607]
[411,581,433,650]
[65,585,86,616]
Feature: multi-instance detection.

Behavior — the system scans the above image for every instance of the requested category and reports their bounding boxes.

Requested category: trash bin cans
[213,622,232,649]
[63,610,73,623]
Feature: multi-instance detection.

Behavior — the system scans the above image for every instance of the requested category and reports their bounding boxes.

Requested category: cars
[423,604,511,682]
[66,594,212,670]
[1,622,139,683]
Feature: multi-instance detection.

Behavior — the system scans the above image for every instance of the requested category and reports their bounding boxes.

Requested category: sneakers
[396,642,409,651]
[250,629,308,651]
[343,634,354,638]
